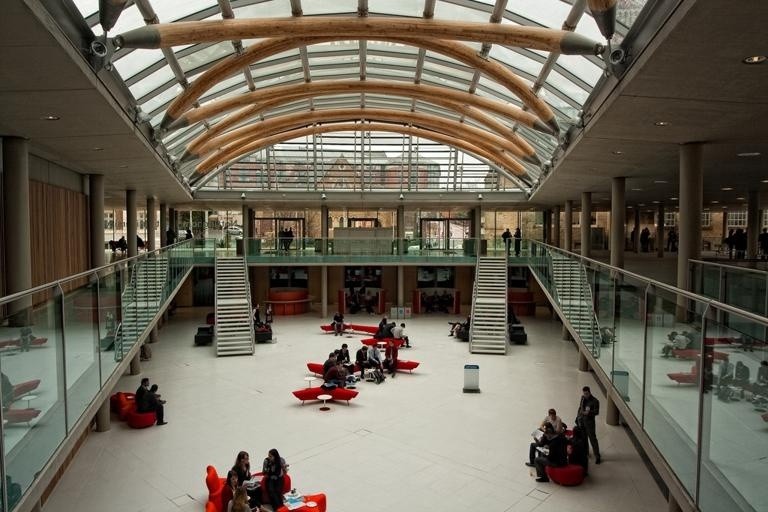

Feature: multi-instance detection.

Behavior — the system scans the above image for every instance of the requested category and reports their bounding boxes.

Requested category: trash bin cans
[315,239,322,252]
[236,239,243,254]
[611,371,630,401]
[391,306,411,319]
[646,314,674,329]
[481,240,487,255]
[249,239,261,256]
[464,238,475,256]
[463,365,480,393]
[404,238,409,253]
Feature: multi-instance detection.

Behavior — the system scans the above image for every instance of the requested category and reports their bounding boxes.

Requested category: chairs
[93,330,117,351]
[110,391,156,429]
[195,326,213,345]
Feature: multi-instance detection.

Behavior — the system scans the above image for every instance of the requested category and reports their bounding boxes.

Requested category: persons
[713,357,734,395]
[725,228,747,260]
[119,236,127,251]
[345,283,378,315]
[279,227,294,250]
[601,326,617,342]
[184,227,192,239]
[661,331,701,359]
[735,361,750,399]
[526,387,601,482]
[664,226,676,253]
[0,372,13,404]
[753,360,768,397]
[501,228,520,255]
[19,327,33,353]
[323,312,412,388]
[253,304,273,332]
[167,224,177,245]
[758,227,768,260]
[631,227,651,252]
[137,235,144,249]
[742,335,754,352]
[448,314,471,337]
[421,289,453,314]
[136,378,168,426]
[223,448,287,512]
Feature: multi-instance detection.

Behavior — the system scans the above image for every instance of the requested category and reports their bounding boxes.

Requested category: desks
[377,342,387,353]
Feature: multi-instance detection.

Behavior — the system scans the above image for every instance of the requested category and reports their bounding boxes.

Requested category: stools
[545,431,584,485]
[253,474,290,503]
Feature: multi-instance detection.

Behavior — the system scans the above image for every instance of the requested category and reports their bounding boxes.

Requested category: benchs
[667,370,768,426]
[339,287,385,315]
[414,288,461,314]
[670,337,765,365]
[1,379,41,428]
[0,337,48,348]
[320,324,404,348]
[292,360,420,407]
